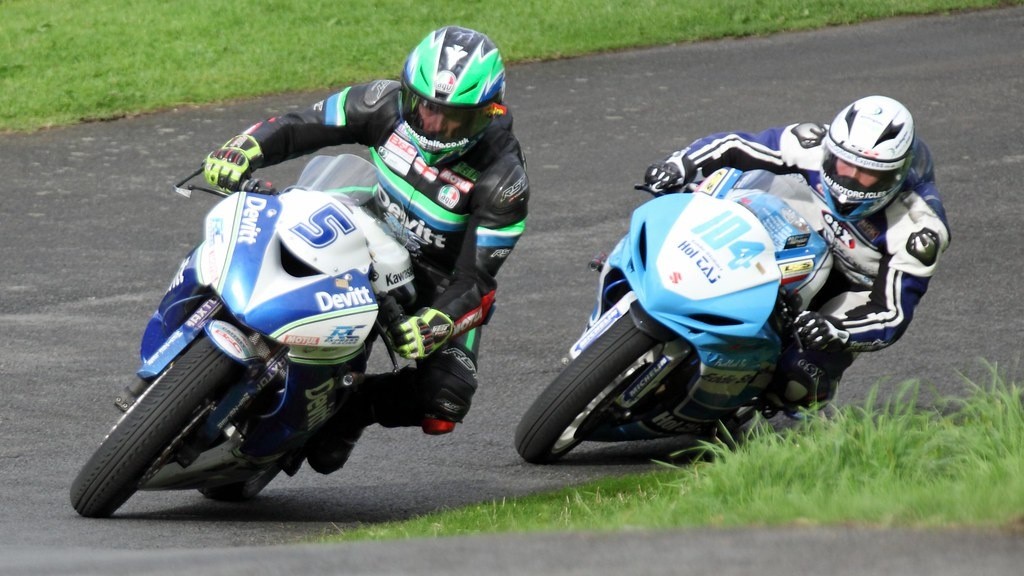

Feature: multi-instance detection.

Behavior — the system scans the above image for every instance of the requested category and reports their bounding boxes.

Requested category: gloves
[385,306,455,359]
[644,151,696,194]
[204,133,264,195]
[793,309,851,355]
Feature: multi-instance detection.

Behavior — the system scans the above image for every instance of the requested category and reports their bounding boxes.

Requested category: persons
[139,25,532,476]
[643,93,953,418]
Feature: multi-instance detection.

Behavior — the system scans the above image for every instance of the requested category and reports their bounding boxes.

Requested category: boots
[306,366,420,473]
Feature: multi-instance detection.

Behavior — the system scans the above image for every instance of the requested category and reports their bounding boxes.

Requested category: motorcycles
[511,167,853,470]
[65,169,489,520]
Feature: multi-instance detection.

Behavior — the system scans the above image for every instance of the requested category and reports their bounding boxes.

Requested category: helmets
[397,25,505,168]
[820,96,915,221]
